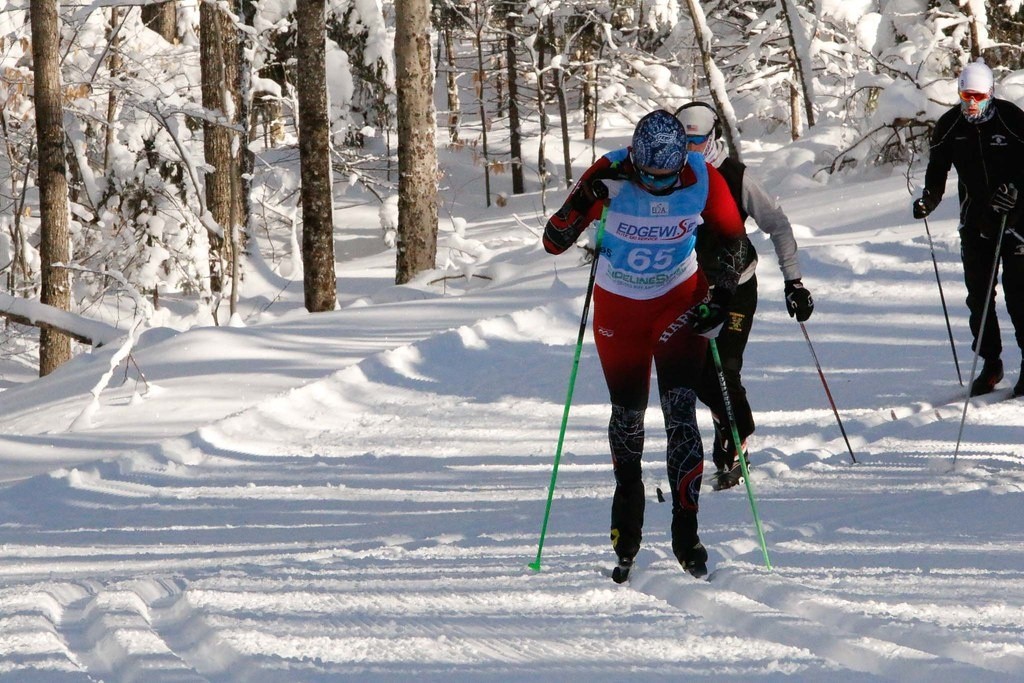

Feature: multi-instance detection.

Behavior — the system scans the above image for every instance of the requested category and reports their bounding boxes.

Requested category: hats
[677,104,717,135]
[631,110,686,169]
[959,56,993,94]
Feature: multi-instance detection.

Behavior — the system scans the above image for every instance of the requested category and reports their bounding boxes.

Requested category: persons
[674,102,815,492]
[542,110,752,585]
[912,62,1024,398]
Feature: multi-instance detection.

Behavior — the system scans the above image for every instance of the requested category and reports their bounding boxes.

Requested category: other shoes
[672,532,708,579]
[611,527,642,583]
[718,457,750,488]
[970,359,1003,397]
[1014,361,1024,395]
[721,385,755,440]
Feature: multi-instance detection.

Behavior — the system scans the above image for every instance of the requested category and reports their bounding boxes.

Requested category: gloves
[785,278,814,322]
[689,288,728,338]
[582,167,628,199]
[991,183,1018,215]
[913,189,940,219]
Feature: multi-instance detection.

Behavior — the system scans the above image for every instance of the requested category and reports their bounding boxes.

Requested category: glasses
[685,124,714,145]
[959,88,992,103]
[633,162,682,191]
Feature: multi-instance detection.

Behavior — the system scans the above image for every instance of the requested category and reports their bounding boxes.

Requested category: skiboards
[656,487,666,502]
[889,409,944,422]
